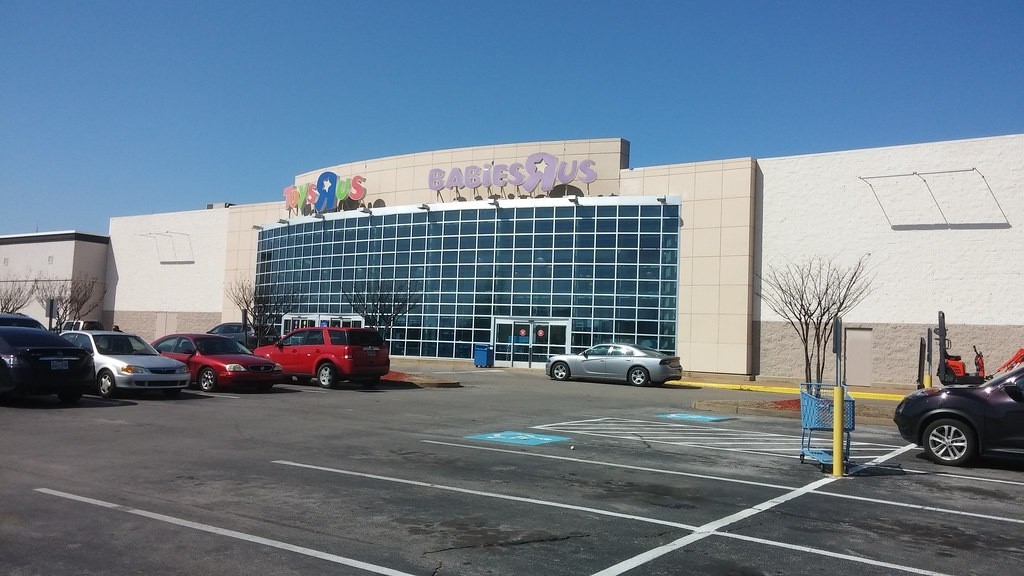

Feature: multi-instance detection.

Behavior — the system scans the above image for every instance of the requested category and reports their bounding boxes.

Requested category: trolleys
[798,382,857,475]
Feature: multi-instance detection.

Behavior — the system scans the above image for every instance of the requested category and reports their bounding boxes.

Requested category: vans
[54,319,105,349]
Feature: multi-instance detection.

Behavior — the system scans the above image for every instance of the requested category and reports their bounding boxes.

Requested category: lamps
[251,225,264,230]
[657,195,666,202]
[487,199,499,206]
[568,195,578,204]
[417,203,429,210]
[312,213,325,220]
[277,218,289,224]
[357,207,372,214]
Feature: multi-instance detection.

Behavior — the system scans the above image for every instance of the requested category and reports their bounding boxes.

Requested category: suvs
[205,321,282,353]
[254,326,390,388]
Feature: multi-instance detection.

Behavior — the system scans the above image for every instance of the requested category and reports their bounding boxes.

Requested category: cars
[59,330,192,400]
[0,327,96,406]
[135,333,285,392]
[0,311,48,332]
[892,364,1024,467]
[544,343,682,387]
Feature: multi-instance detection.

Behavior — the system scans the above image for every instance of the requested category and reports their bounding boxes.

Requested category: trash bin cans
[474,343,494,368]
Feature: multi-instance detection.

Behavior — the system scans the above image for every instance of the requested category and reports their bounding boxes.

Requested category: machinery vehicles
[915,311,1024,387]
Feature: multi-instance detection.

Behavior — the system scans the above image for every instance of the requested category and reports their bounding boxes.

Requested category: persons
[107,325,133,352]
